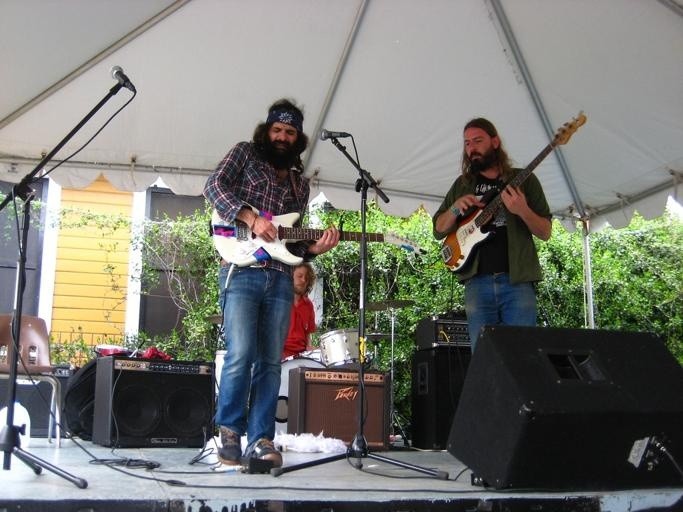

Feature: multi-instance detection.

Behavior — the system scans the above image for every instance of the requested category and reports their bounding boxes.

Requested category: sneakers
[217,427,282,466]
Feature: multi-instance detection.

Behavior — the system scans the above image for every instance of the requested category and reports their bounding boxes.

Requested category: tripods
[0,87,124,488]
[270,142,450,480]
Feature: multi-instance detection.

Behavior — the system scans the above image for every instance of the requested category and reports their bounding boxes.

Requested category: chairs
[0,312,62,449]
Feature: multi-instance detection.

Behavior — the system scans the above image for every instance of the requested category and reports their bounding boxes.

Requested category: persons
[280,264,316,364]
[432,119,552,354]
[205,99,339,467]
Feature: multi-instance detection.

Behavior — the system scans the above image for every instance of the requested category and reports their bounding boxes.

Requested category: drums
[284,350,323,365]
[275,353,330,433]
[319,328,374,373]
[97,344,131,356]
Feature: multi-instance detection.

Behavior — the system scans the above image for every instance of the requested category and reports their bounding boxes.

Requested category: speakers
[288,368,390,451]
[411,348,472,449]
[93,357,215,449]
[447,326,683,490]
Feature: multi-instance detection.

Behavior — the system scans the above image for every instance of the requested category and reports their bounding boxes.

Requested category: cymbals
[203,314,223,324]
[360,301,414,312]
[366,333,398,339]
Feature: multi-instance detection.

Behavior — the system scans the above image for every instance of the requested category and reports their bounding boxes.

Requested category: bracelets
[448,203,462,217]
[250,214,257,231]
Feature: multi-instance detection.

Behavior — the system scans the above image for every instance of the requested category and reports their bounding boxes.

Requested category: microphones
[111,66,135,91]
[320,129,350,140]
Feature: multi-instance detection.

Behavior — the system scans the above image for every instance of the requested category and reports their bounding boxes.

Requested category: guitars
[210,200,428,267]
[441,111,586,274]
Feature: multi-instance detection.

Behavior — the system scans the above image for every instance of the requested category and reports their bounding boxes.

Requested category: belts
[220,257,285,273]
